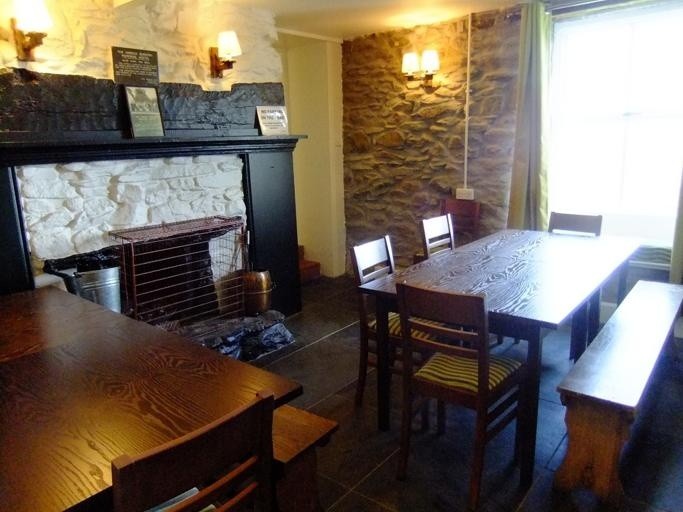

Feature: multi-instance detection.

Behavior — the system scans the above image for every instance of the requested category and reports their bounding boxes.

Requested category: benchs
[270,403,338,511]
[554,278,683,511]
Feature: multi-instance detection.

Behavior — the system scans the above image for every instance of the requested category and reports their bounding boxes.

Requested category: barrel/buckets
[73,267,123,314]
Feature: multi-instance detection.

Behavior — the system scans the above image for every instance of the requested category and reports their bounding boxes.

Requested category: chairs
[393,280,524,511]
[420,213,521,345]
[550,211,602,362]
[350,234,464,436]
[110,389,276,510]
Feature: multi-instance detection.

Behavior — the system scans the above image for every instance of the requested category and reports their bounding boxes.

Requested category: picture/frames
[124,85,166,139]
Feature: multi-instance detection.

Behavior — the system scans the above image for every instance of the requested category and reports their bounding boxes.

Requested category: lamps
[400,49,439,89]
[11,0,51,61]
[207,30,241,78]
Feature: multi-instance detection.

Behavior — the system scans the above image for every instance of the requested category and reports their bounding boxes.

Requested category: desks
[358,227,642,493]
[1,286,306,511]
